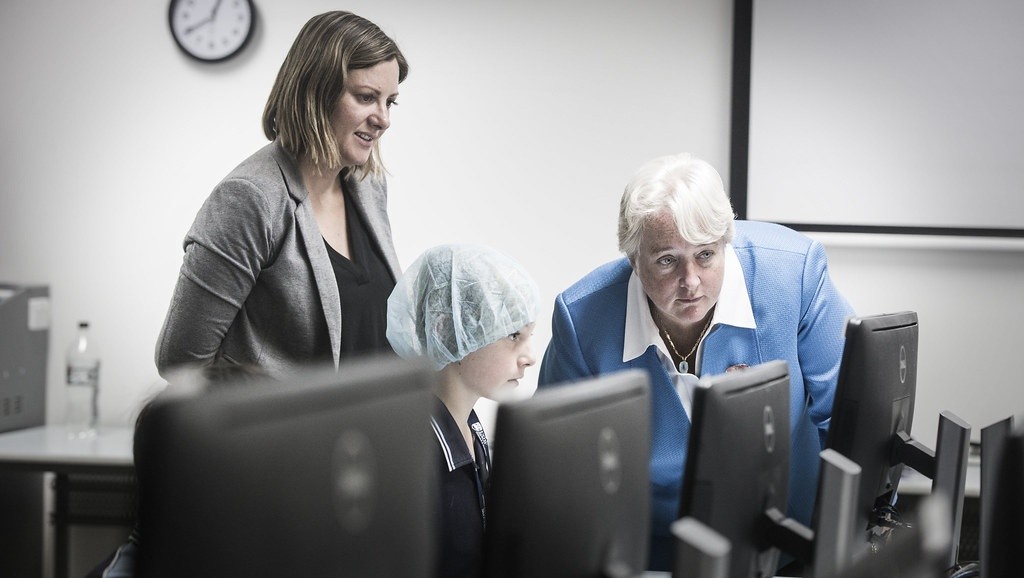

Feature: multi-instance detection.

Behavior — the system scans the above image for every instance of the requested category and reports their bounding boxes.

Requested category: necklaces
[652,306,714,374]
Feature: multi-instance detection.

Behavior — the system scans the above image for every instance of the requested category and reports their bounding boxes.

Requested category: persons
[154,11,409,393]
[385,241,535,565]
[530,154,862,502]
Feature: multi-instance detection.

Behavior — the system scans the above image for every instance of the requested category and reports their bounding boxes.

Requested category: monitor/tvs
[132,311,972,578]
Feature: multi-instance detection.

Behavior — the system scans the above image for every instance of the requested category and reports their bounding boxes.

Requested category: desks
[0,421,134,578]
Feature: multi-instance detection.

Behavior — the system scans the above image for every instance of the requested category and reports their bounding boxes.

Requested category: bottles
[62,319,100,447]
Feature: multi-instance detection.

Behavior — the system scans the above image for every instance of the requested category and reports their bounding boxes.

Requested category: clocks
[168,0,255,64]
